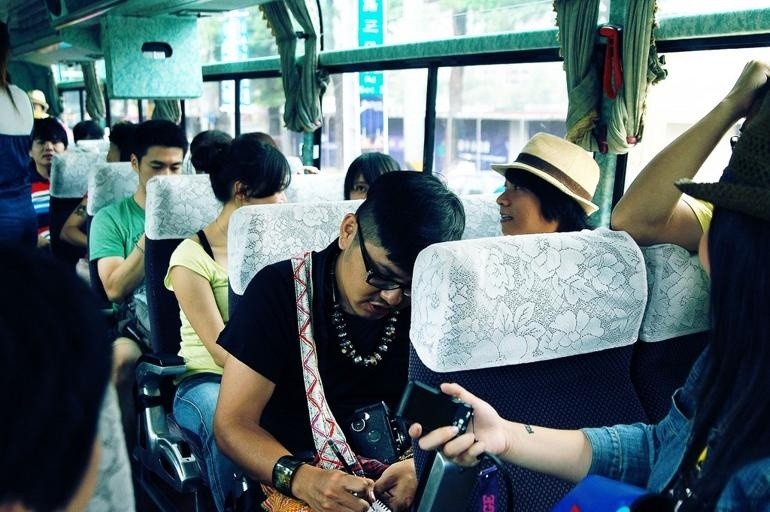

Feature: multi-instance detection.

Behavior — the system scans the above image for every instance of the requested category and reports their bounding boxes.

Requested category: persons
[491,130,601,236]
[1,23,34,136]
[1,91,188,512]
[408,59,770,512]
[217,171,466,512]
[343,152,401,200]
[163,129,291,511]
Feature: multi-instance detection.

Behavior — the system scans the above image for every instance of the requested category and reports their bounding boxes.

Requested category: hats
[27,90,49,112]
[491,132,601,216]
[674,91,770,221]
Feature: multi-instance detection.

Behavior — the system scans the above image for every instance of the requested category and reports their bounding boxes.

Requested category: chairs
[49,137,711,512]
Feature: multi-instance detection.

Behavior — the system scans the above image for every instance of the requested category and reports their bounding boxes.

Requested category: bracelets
[272,455,306,499]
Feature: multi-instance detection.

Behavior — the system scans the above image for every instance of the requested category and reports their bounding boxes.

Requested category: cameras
[349,401,412,464]
[396,379,473,440]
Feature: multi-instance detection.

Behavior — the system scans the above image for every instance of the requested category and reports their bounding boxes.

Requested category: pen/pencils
[327,440,375,511]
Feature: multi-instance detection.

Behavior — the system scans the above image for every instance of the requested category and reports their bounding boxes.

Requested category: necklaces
[333,271,400,368]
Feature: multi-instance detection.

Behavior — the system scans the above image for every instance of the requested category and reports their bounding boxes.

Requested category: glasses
[355,212,411,299]
